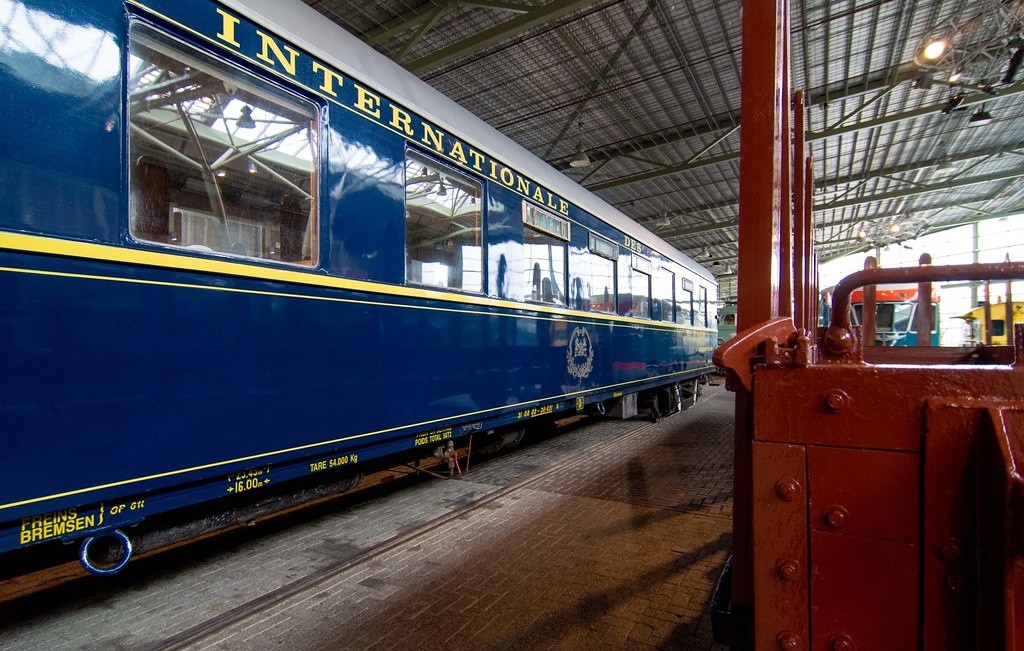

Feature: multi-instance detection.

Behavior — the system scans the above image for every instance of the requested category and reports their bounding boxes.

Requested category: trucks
[820,279,942,346]
[950,302,1024,351]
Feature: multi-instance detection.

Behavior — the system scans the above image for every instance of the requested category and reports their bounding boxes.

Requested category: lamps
[470,198,477,207]
[421,167,427,177]
[236,92,256,128]
[217,166,227,177]
[447,239,454,249]
[436,182,448,196]
[248,161,258,173]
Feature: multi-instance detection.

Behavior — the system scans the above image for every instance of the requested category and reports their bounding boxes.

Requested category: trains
[1,0,722,603]
